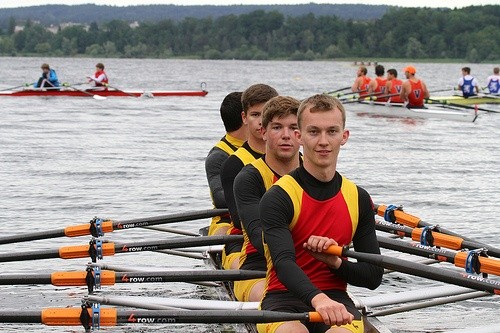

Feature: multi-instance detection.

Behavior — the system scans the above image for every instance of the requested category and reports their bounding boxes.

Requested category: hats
[401,65,416,74]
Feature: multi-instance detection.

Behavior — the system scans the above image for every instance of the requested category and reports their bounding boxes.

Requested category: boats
[337,96,479,123]
[198,224,393,333]
[0,80,209,97]
[427,93,500,106]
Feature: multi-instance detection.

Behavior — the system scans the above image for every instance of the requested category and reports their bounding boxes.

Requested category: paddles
[0,199,500,296]
[0,304,323,326]
[318,81,499,122]
[0,271,269,287]
[0,75,134,102]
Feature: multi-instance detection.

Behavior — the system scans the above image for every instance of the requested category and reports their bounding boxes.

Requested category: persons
[352,67,371,98]
[86,63,108,91]
[205,83,304,303]
[457,67,479,98]
[257,95,384,333]
[399,66,429,109]
[368,65,387,105]
[384,69,405,107]
[486,67,500,97]
[34,64,60,90]
